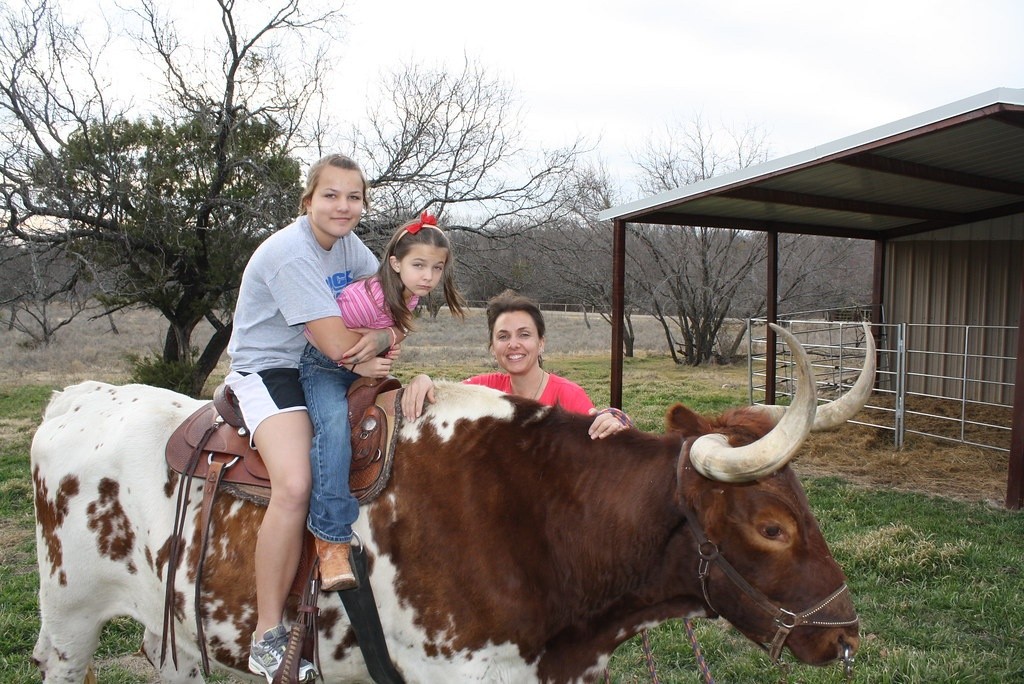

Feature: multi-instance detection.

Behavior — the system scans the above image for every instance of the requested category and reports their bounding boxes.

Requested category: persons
[401,289,636,440]
[297,209,471,592]
[224,153,413,684]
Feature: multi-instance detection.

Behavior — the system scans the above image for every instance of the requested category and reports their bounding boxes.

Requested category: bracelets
[350,364,356,373]
[387,327,397,350]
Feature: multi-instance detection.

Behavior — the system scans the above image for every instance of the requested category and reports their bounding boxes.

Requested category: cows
[30,320,877,684]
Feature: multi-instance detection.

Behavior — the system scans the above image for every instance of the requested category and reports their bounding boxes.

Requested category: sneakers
[315,536,359,592]
[249,625,318,684]
